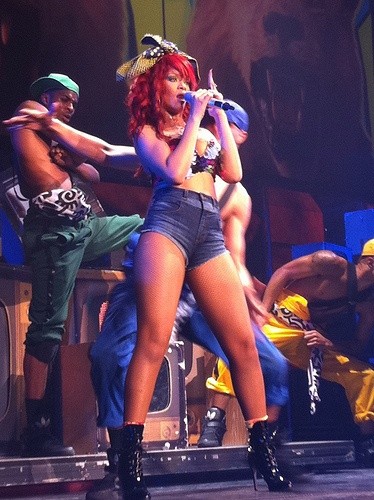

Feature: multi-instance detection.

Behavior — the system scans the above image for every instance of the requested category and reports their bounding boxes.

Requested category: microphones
[183,91,235,111]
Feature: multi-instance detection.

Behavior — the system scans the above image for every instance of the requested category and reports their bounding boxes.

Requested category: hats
[361,238,374,256]
[29,73,80,98]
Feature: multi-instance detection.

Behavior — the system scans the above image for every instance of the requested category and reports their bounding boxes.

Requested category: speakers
[62,339,188,453]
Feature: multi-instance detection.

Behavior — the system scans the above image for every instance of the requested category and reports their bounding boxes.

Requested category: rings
[208,83,217,89]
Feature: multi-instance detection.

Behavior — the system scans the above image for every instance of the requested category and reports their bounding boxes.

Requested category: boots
[247,419,294,492]
[109,423,152,500]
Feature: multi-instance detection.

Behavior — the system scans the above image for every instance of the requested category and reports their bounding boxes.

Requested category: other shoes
[198,408,227,447]
[355,435,374,468]
[86,448,120,500]
[22,416,75,457]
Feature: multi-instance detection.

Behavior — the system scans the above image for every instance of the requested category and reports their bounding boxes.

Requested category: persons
[2,74,374,500]
[116,33,292,500]
